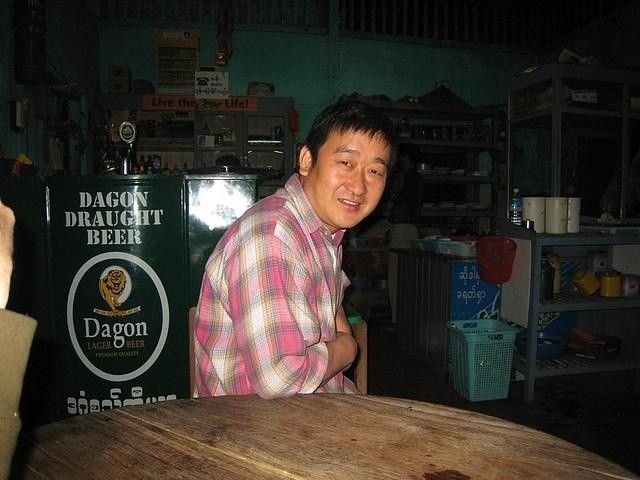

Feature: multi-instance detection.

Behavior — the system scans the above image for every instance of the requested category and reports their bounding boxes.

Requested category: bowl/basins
[516,337,569,360]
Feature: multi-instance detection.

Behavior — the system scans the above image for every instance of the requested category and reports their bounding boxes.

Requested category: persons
[192,99,400,400]
[378,148,426,333]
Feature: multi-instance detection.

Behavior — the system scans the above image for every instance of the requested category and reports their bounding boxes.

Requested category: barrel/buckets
[46,174,189,422]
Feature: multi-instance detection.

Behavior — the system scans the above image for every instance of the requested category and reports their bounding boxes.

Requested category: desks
[7,393,639,480]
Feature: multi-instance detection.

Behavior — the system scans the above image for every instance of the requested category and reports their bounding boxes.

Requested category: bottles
[509,188,523,226]
[133,154,189,175]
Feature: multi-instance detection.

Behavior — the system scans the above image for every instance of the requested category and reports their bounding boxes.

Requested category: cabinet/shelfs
[507,70,635,229]
[506,229,639,403]
[341,97,498,242]
[91,93,294,203]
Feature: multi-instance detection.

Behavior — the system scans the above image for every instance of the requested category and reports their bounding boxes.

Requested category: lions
[98,268,127,319]
